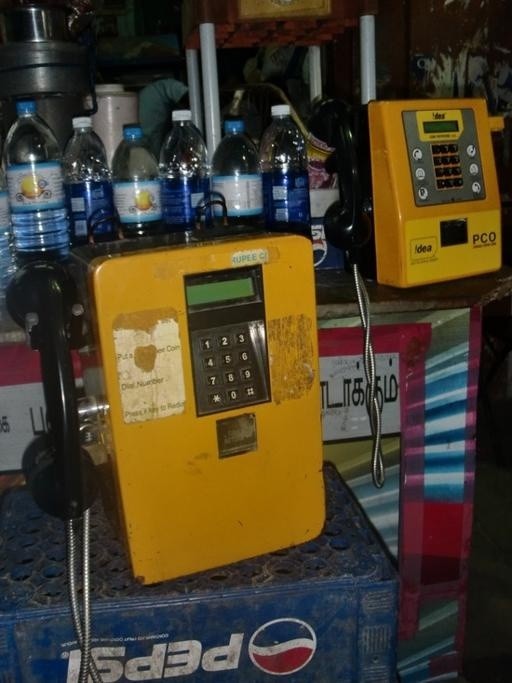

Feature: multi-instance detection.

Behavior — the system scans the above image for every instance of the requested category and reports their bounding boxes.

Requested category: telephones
[8,235,326,584]
[313,93,502,287]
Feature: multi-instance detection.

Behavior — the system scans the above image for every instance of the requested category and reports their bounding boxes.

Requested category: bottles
[63,109,115,251]
[261,100,314,244]
[113,119,161,238]
[5,97,73,258]
[0,162,20,305]
[207,114,263,236]
[159,106,210,234]
